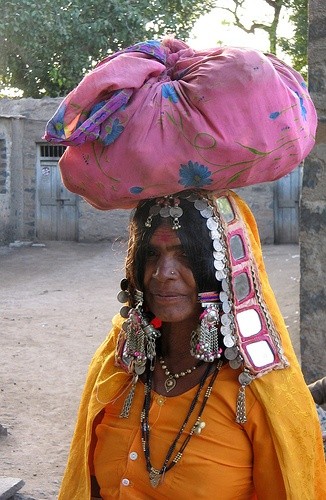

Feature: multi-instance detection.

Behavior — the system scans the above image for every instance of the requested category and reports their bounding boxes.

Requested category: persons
[58,189,326,500]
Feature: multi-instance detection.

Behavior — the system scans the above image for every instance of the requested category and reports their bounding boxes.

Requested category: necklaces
[156,351,206,394]
[137,347,225,490]
